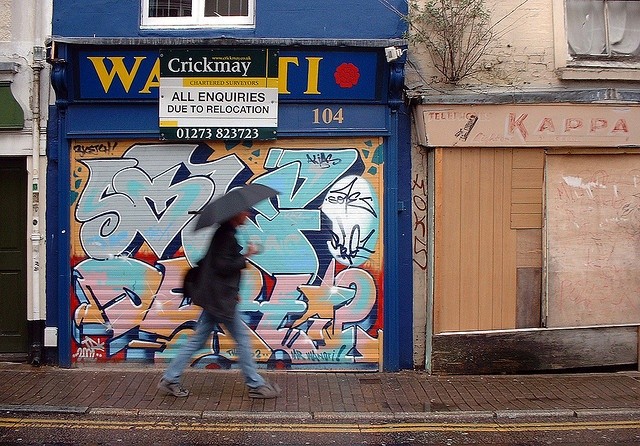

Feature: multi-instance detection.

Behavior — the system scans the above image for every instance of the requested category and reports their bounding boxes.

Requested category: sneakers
[249,385,282,399]
[158,376,190,397]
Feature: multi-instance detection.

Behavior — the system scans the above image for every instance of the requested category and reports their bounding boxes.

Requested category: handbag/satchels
[184,267,202,296]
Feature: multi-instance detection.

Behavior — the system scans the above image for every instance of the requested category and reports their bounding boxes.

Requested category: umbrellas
[194,182,281,255]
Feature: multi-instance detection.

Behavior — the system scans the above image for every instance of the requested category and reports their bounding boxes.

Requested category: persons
[157,211,284,399]
